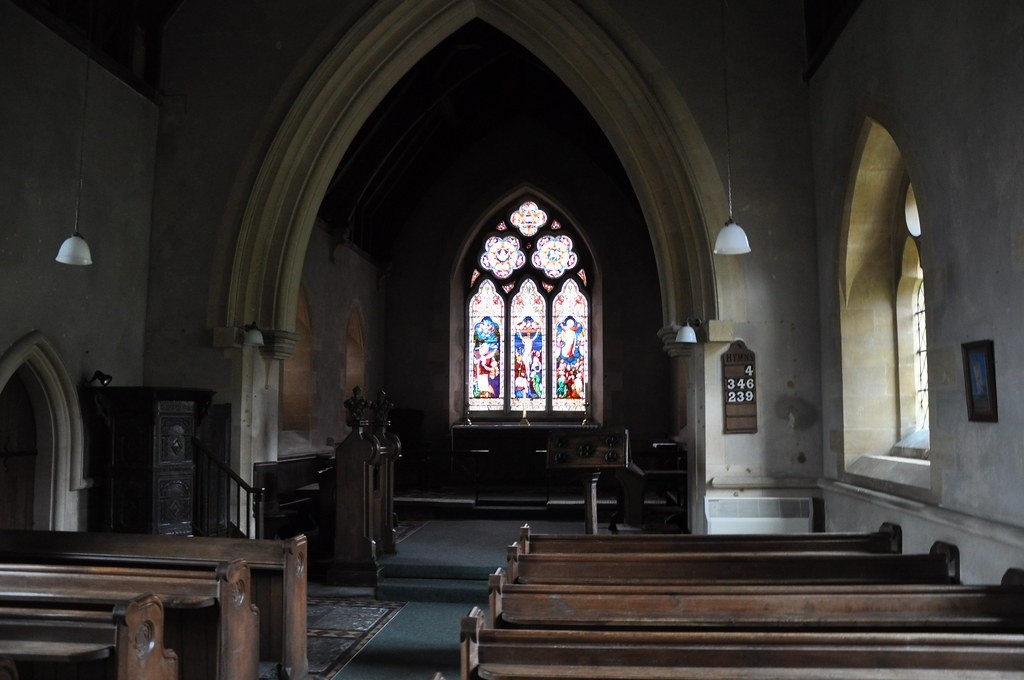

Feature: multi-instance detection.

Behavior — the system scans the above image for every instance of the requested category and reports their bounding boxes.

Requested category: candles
[523,390,527,418]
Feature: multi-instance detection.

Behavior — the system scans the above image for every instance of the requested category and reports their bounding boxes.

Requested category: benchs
[430,520,1024,680]
[0,528,310,680]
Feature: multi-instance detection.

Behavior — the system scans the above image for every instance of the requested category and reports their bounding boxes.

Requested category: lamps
[53,0,93,266]
[712,0,752,256]
[674,317,702,343]
[239,320,264,347]
[86,370,113,386]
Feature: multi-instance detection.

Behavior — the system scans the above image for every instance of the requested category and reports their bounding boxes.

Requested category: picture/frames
[960,339,998,422]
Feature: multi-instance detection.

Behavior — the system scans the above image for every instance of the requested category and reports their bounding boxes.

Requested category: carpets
[259,595,409,680]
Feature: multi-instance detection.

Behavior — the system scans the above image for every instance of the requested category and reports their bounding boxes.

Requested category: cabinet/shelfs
[624,442,687,533]
